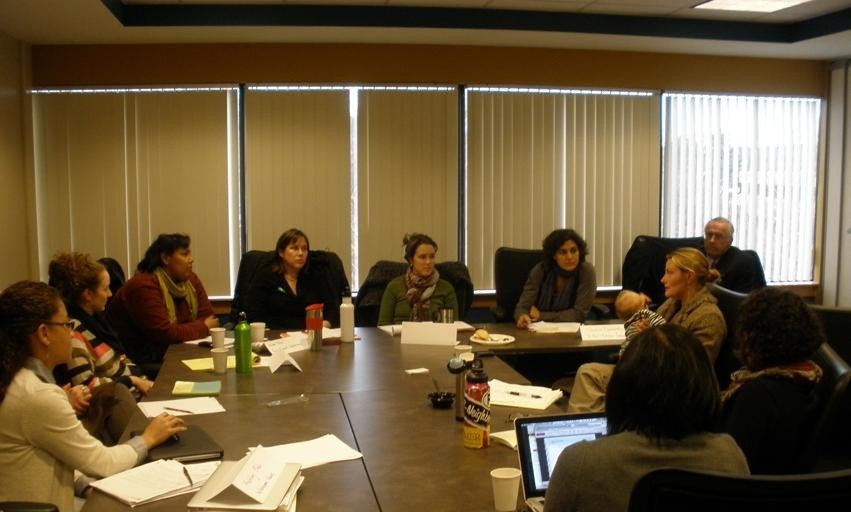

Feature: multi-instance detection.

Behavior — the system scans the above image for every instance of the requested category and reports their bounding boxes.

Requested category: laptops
[514,411,610,512]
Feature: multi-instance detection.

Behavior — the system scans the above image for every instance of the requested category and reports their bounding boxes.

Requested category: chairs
[98,259,166,370]
[742,251,766,290]
[628,467,851,512]
[231,249,353,328]
[490,247,610,321]
[355,261,475,326]
[818,343,851,388]
[621,235,706,308]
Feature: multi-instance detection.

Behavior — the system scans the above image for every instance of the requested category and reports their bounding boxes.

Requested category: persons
[40,250,156,397]
[513,228,597,330]
[544,218,832,512]
[50,382,148,447]
[372,232,461,323]
[238,228,340,331]
[100,233,220,381]
[1,280,188,512]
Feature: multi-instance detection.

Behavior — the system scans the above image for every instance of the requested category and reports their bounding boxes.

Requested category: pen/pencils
[183,466,193,487]
[163,407,193,413]
[507,392,541,398]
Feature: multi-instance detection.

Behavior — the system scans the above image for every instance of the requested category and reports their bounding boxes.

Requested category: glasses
[44,320,75,330]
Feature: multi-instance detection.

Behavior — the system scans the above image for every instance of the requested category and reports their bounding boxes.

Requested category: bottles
[235,312,252,374]
[448,345,492,449]
[340,287,354,343]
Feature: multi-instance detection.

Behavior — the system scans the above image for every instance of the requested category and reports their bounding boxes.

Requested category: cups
[305,304,324,351]
[490,467,521,511]
[211,348,229,374]
[209,328,226,348]
[432,307,454,323]
[250,322,265,342]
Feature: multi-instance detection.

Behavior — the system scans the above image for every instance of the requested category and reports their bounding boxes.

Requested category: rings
[82,387,88,393]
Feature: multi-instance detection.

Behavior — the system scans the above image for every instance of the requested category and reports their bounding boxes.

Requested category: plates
[425,392,456,409]
[470,334,516,345]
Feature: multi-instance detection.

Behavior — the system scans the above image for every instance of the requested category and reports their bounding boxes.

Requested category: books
[424,319,476,331]
[182,352,262,370]
[122,421,225,465]
[491,429,519,450]
[488,378,565,410]
[528,321,581,333]
[376,323,402,337]
[90,456,306,512]
[172,379,222,395]
[280,329,362,343]
